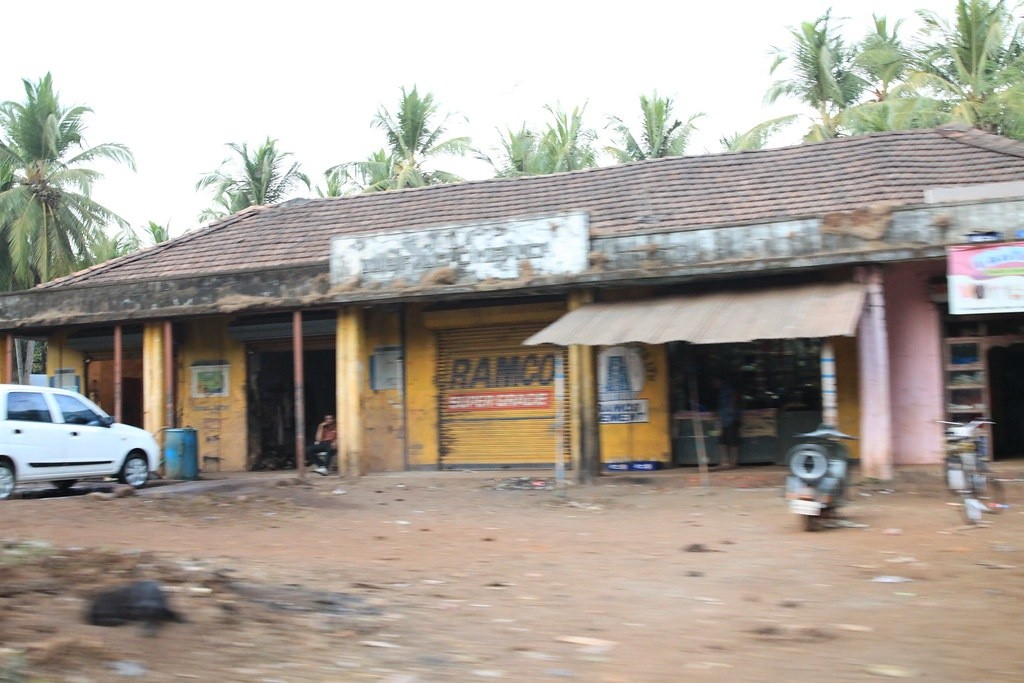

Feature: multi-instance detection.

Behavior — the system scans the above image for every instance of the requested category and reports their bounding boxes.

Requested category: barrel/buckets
[163,427,198,479]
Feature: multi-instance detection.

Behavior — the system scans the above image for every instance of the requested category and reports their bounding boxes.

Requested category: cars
[0,382,161,501]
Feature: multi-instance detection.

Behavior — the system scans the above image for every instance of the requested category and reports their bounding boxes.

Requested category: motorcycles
[785,424,860,532]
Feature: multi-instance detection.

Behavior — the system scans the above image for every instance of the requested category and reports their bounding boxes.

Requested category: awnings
[522,283,868,346]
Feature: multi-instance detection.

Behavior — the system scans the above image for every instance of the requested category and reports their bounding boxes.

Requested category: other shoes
[717,463,739,470]
[312,466,329,475]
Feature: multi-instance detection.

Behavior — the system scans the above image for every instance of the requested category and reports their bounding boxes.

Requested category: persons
[304,413,337,474]
[712,374,739,468]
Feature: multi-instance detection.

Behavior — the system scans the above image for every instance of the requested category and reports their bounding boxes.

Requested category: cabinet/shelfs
[943,336,992,464]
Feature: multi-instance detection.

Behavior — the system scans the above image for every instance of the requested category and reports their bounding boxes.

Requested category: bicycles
[943,417,1008,524]
